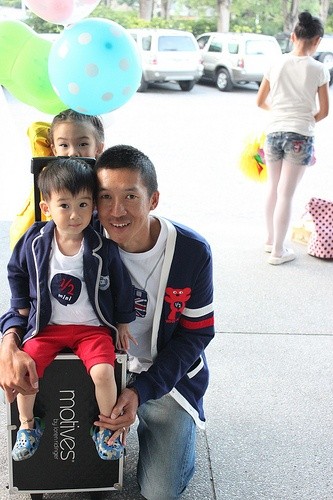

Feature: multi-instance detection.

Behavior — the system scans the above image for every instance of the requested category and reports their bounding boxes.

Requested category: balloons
[0,19,73,117]
[27,1,102,28]
[47,16,143,115]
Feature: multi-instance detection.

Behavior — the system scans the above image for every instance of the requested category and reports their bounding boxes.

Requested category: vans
[120,29,203,92]
[195,32,285,92]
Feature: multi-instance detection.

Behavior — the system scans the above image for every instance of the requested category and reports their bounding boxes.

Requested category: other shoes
[269,248,296,264]
[264,245,273,252]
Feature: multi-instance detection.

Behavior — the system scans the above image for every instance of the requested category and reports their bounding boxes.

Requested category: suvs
[282,35,333,87]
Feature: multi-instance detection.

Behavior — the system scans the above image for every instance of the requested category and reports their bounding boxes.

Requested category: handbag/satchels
[302,197,333,259]
[239,130,266,179]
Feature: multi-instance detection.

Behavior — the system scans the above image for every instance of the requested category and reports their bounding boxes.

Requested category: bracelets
[0,330,22,347]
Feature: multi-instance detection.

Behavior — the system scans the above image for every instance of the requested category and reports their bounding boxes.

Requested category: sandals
[90,423,124,460]
[12,417,45,461]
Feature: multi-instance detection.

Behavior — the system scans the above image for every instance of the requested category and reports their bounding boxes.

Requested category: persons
[5,158,138,464]
[49,108,105,167]
[91,143,215,500]
[254,11,331,266]
